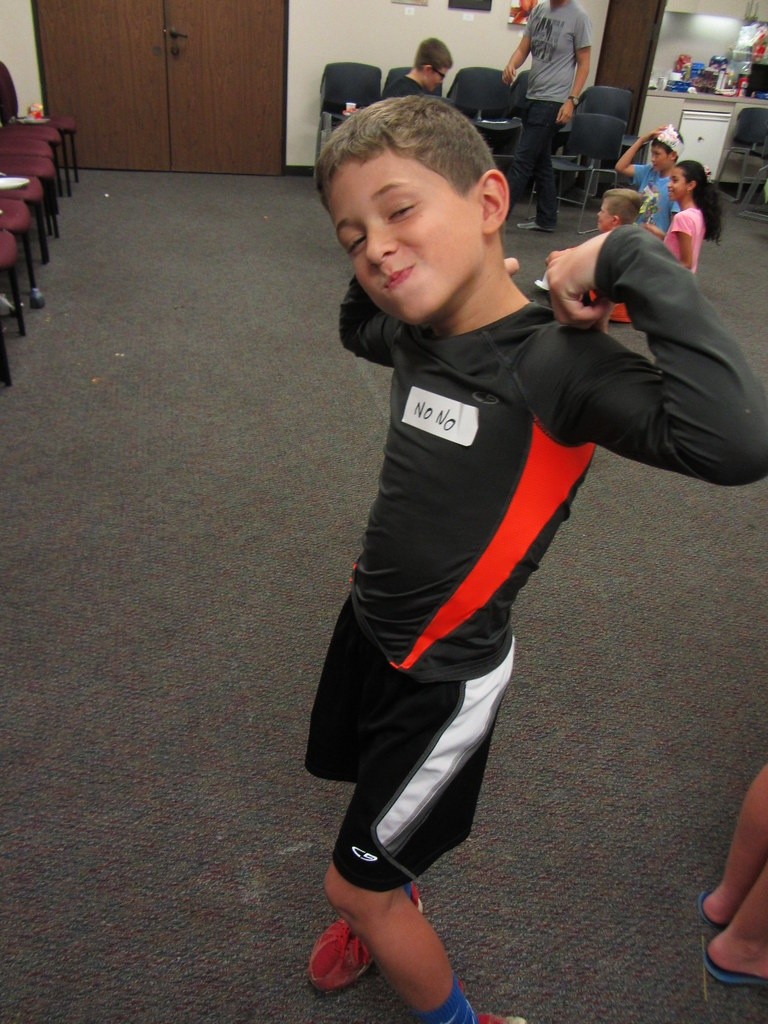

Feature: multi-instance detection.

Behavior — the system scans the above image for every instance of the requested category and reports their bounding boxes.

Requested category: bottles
[713,67,751,97]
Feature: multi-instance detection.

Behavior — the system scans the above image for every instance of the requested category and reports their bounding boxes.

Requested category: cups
[670,71,681,80]
[346,103,356,110]
[656,77,667,91]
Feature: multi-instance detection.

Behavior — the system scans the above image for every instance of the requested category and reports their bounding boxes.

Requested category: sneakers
[477,1013,526,1024]
[309,883,424,992]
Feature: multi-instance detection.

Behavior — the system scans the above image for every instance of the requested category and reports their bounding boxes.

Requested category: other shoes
[516,222,556,232]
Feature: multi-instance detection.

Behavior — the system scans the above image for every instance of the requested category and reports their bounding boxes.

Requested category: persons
[546,189,642,322]
[616,123,684,233]
[502,0,592,231]
[642,160,725,273]
[383,37,452,99]
[308,96,768,1024]
[697,764,768,985]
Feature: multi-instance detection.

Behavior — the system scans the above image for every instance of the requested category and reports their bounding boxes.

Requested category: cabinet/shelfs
[636,89,768,184]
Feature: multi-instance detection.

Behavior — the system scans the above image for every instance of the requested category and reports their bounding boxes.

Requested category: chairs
[578,86,650,188]
[510,70,574,197]
[0,61,79,385]
[382,67,442,100]
[527,114,627,235]
[445,67,522,158]
[313,62,381,188]
[712,107,768,224]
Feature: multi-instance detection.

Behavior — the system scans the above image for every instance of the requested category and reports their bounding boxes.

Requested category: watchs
[567,96,579,105]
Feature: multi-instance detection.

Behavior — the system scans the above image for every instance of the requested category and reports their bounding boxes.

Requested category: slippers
[697,888,729,928]
[703,948,766,986]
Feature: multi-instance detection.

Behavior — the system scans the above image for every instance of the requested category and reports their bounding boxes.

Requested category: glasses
[428,67,446,81]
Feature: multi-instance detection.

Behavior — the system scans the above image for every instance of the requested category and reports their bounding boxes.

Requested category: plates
[23,118,50,124]
[0,177,31,189]
